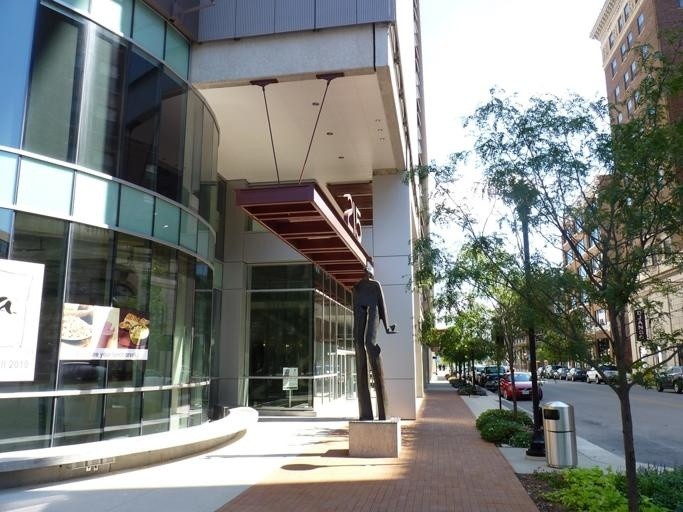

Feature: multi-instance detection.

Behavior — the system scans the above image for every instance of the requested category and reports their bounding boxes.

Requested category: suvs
[656,366,683,393]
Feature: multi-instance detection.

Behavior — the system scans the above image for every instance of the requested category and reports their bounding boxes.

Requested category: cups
[128,324,150,349]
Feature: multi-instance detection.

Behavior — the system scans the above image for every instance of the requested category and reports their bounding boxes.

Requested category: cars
[457,364,630,400]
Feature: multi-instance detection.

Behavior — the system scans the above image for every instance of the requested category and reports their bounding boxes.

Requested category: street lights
[512,184,545,457]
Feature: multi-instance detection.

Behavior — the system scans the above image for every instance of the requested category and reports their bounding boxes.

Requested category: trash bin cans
[541,401,578,470]
